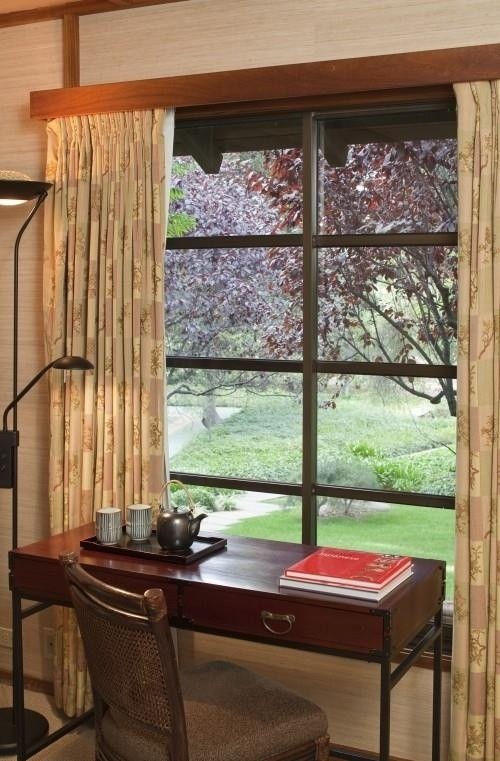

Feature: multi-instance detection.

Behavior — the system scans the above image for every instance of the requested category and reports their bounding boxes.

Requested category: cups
[94,507,123,548]
[124,503,153,544]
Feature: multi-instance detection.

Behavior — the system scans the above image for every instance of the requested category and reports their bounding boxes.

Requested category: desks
[10,521,447,761]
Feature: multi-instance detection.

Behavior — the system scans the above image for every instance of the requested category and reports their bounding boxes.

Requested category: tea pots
[154,479,209,553]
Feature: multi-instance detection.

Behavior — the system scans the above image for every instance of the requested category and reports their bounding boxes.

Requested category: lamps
[0,166,96,756]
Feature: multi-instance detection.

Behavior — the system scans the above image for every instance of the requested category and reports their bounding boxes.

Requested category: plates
[78,523,230,566]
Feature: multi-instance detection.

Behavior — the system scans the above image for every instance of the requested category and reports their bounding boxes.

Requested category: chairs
[60,555,335,761]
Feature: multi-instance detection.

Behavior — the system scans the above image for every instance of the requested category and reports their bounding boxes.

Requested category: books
[279,564,415,602]
[284,547,412,590]
